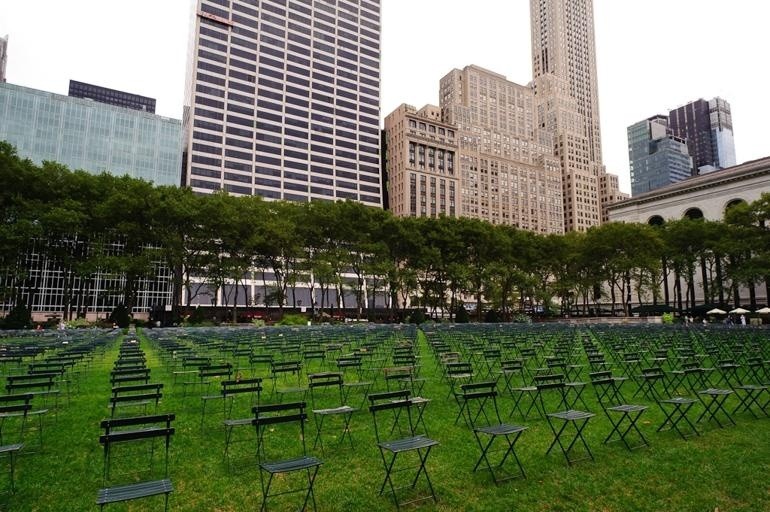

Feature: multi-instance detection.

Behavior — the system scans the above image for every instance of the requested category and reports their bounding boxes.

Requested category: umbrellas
[729,308,750,314]
[705,308,726,315]
[756,307,770,313]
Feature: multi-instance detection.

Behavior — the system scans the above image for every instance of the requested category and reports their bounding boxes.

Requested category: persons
[740,314,747,326]
[684,314,689,327]
[702,318,708,325]
[689,316,694,324]
[726,315,733,328]
